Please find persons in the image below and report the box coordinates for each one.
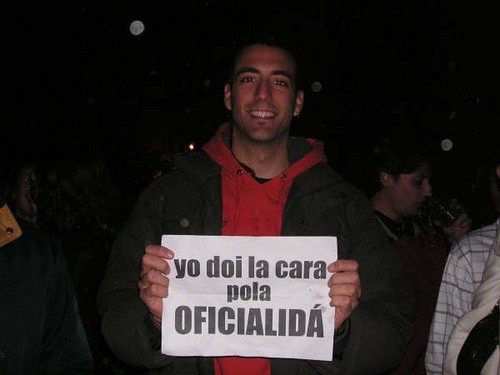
[96,28,416,375]
[363,135,472,375]
[0,201,95,375]
[424,218,500,375]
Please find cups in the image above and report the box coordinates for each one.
[424,196,458,228]
